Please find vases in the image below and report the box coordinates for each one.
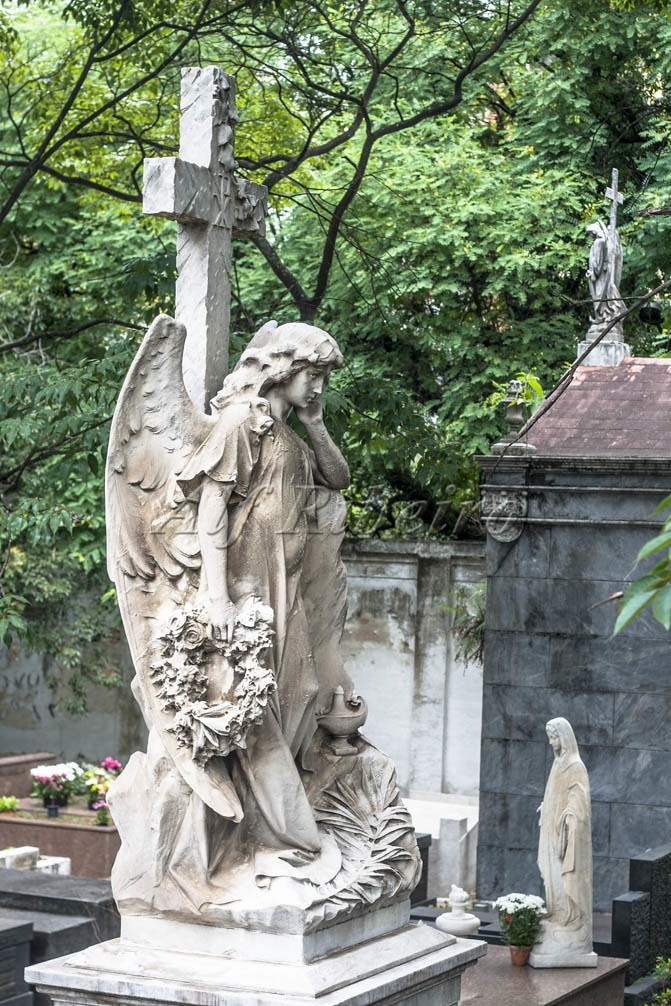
[44,793,68,809]
[510,945,532,968]
[88,800,96,809]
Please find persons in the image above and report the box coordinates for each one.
[587,221,622,321]
[175,321,353,876]
[536,716,593,954]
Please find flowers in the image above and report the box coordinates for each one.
[492,892,552,948]
[29,756,123,803]
